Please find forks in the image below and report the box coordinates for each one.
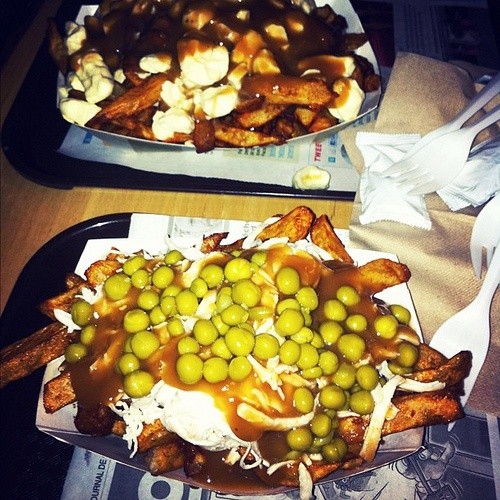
[469,192,500,279]
[428,249,500,432]
[380,79,500,195]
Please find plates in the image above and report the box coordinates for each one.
[56,0,381,149]
[34,238,423,493]
